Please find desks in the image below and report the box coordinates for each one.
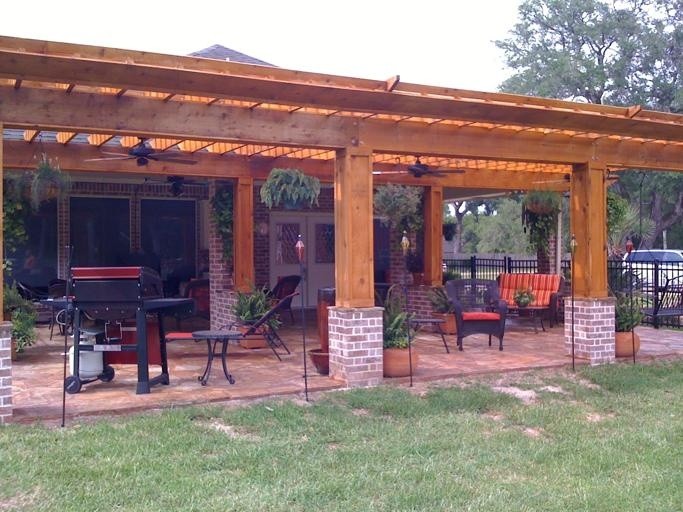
[39,294,72,340]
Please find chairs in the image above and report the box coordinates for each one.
[17,283,55,340]
[264,274,299,324]
[639,276,682,328]
[444,277,507,352]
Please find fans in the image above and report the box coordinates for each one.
[82,132,199,168]
[368,156,466,178]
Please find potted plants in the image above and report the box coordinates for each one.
[259,167,321,211]
[375,285,456,377]
[616,291,645,357]
[223,280,282,349]
[522,171,561,232]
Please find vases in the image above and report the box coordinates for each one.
[308,349,329,374]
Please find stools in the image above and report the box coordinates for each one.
[193,329,244,385]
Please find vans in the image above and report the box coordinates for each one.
[616,249,683,293]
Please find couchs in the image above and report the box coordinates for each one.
[493,273,562,329]
